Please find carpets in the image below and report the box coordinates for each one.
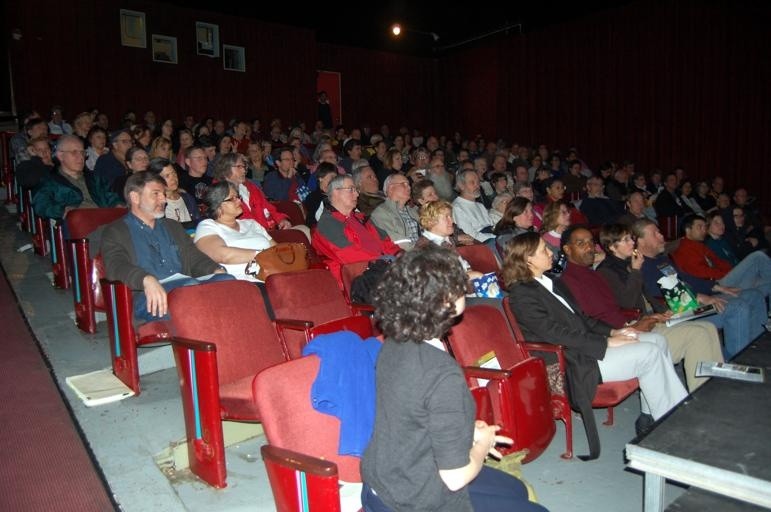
[1,262,124,512]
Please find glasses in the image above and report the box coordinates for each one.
[224,194,242,202]
[337,186,361,193]
[131,156,149,161]
[61,149,85,156]
[163,171,178,180]
[231,165,249,169]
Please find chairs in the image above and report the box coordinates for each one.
[48,217,71,290]
[253,343,376,512]
[30,202,49,257]
[67,207,129,335]
[340,261,375,316]
[167,280,313,489]
[457,244,504,288]
[92,226,173,394]
[0,136,30,229]
[502,295,639,459]
[447,305,573,465]
[265,268,376,360]
[270,200,305,226]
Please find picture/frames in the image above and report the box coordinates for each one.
[119,8,147,48]
[196,21,220,58]
[223,43,246,72]
[152,34,178,64]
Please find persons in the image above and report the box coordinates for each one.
[192,180,276,284]
[703,211,739,265]
[722,207,771,256]
[360,245,548,512]
[692,181,716,209]
[73,112,90,146]
[733,188,752,207]
[312,174,404,264]
[595,223,670,319]
[559,226,727,393]
[115,108,176,174]
[535,178,579,220]
[82,126,109,167]
[490,192,514,210]
[503,231,686,433]
[599,162,613,179]
[674,214,771,296]
[148,160,205,231]
[301,160,339,219]
[717,194,730,209]
[371,173,423,250]
[656,172,696,218]
[580,176,624,222]
[411,179,475,247]
[618,191,653,226]
[627,173,656,196]
[263,150,303,200]
[629,217,764,358]
[677,177,707,217]
[623,160,636,175]
[15,134,59,189]
[180,145,214,192]
[540,201,571,249]
[608,169,630,199]
[215,154,292,229]
[176,118,339,167]
[8,111,40,156]
[89,106,99,123]
[451,168,494,241]
[674,166,688,185]
[95,113,110,132]
[513,179,543,227]
[33,134,127,220]
[351,166,386,214]
[47,106,70,134]
[335,122,591,178]
[107,147,149,198]
[709,176,724,199]
[317,90,333,130]
[414,199,458,255]
[493,197,534,259]
[100,171,235,321]
[15,120,55,163]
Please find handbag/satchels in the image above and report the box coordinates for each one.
[483,448,538,503]
[350,257,392,316]
[245,241,311,281]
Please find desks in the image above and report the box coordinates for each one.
[626,329,770,512]
